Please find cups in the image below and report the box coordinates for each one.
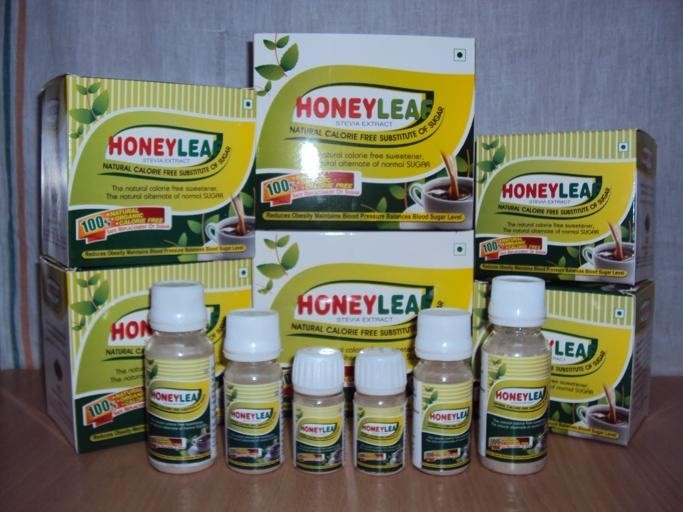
[205,215,256,260]
[575,404,629,445]
[582,241,635,284]
[408,176,474,230]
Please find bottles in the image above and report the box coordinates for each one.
[353,346,407,476]
[144,279,218,474]
[479,275,551,474]
[411,308,474,476]
[223,308,285,475]
[291,345,346,474]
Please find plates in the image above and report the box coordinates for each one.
[197,240,223,261]
[567,421,592,439]
[399,203,439,230]
[574,262,601,282]
